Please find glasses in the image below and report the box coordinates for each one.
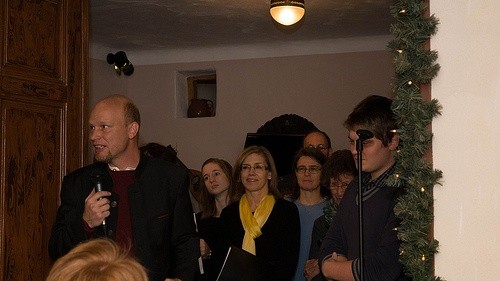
[296,166,320,174]
[329,182,349,190]
[241,163,270,172]
[306,144,329,152]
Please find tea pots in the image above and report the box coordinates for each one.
[187,99,214,118]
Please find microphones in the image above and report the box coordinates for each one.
[93,173,107,231]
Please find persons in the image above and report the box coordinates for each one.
[280,130,332,202]
[293,147,331,281]
[195,158,243,281]
[318,95,410,281]
[47,238,148,281]
[305,150,358,281]
[49,95,200,281]
[218,146,300,281]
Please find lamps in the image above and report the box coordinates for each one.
[269,0,306,27]
